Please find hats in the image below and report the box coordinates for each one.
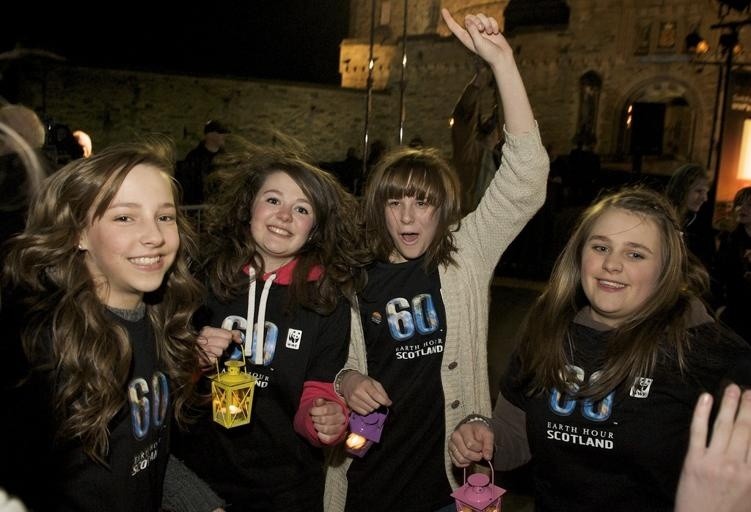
[203,118,232,134]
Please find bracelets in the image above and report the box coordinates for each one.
[335,369,351,392]
[463,419,486,425]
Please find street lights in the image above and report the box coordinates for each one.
[689,29,749,231]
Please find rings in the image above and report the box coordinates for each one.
[466,442,475,448]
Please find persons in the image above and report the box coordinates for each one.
[368,139,385,165]
[556,133,591,169]
[321,9,552,512]
[0,103,62,235]
[179,118,230,206]
[711,187,751,305]
[719,274,751,344]
[447,173,751,512]
[0,133,228,512]
[670,163,714,260]
[171,145,382,511]
